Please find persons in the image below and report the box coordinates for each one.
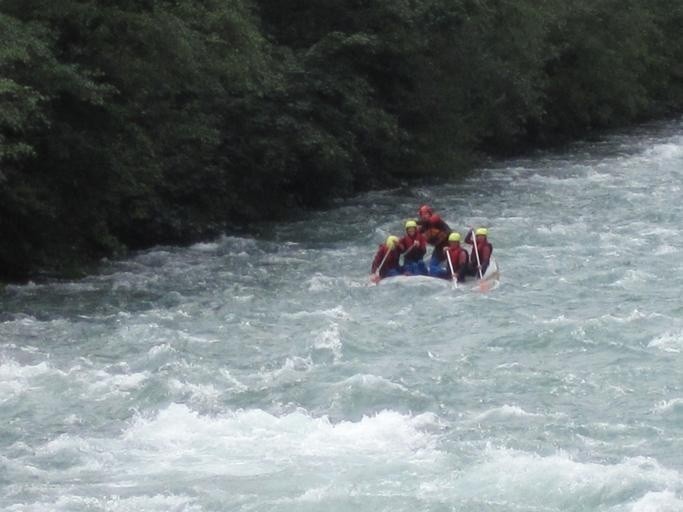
[372,205,493,282]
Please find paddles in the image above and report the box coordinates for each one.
[470,223,489,293]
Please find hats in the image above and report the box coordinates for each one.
[475,228,488,236]
[405,221,417,229]
[418,205,442,225]
[448,232,461,242]
[386,235,399,248]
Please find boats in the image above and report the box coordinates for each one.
[368,245,502,294]
